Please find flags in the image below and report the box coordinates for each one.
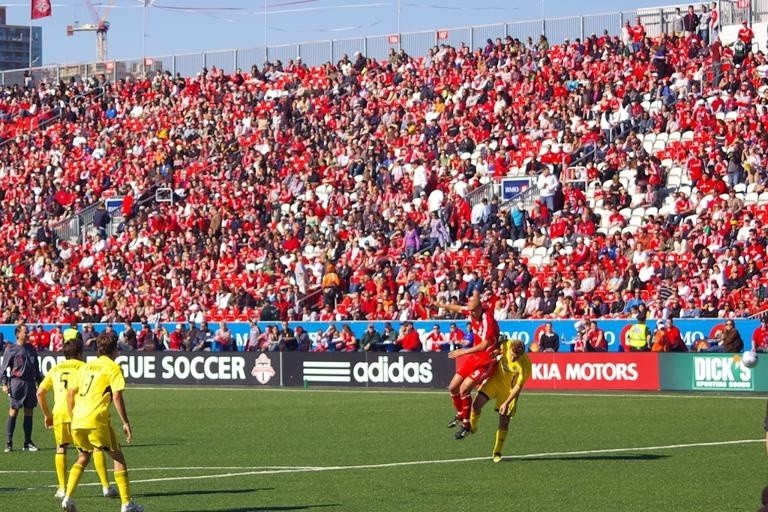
[32,0,51,20]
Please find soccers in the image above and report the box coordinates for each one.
[742,351,758,367]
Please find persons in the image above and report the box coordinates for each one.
[36,339,120,498]
[0,324,43,452]
[60,333,143,512]
[0,2,768,354]
[430,299,499,440]
[469,339,532,462]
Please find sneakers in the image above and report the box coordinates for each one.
[454,425,471,440]
[3,440,12,452]
[470,406,480,434]
[492,450,502,463]
[22,441,39,452]
[60,495,76,511]
[101,484,119,497]
[54,488,65,497]
[121,498,145,512]
[446,414,463,428]
